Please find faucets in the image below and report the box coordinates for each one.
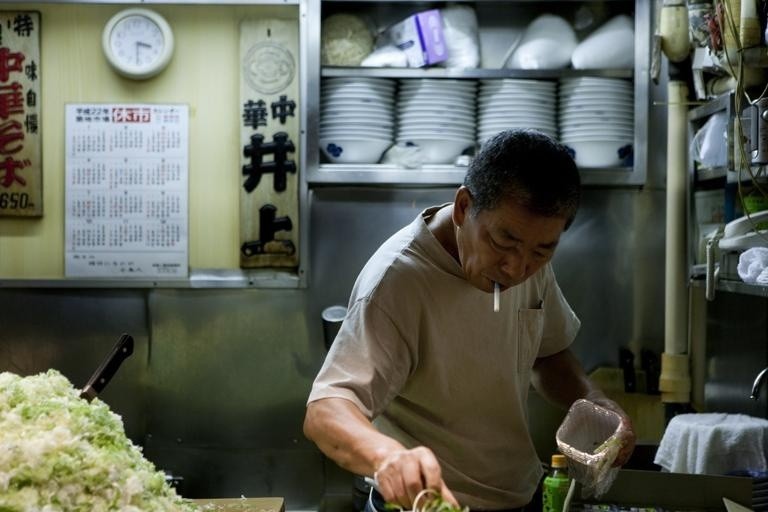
[748,368,767,394]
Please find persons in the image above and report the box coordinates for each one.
[303,126,638,510]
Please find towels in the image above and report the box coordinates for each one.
[653,412,768,475]
[738,248,768,286]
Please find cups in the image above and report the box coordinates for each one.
[554,396,624,497]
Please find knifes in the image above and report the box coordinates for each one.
[616,347,660,397]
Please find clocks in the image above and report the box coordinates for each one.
[103,8,175,81]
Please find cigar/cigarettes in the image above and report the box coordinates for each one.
[493,281,500,312]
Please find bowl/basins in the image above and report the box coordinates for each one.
[318,74,633,170]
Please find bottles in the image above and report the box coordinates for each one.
[543,455,577,512]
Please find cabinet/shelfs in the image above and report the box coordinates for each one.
[302,1,649,187]
[685,95,768,296]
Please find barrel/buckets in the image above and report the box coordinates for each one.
[390,10,450,67]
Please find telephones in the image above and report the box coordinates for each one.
[718,210,768,251]
